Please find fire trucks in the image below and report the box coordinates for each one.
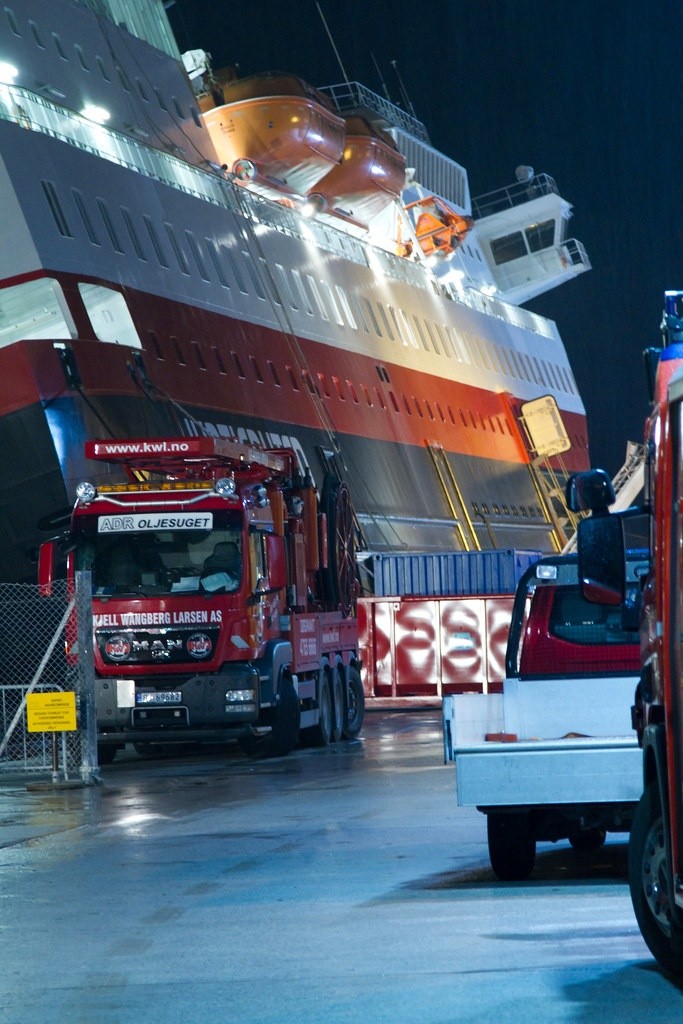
[38,434,370,776]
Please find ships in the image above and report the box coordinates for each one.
[0,0,587,713]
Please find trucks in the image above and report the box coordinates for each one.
[446,514,661,877]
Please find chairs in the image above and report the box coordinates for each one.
[203,541,241,578]
